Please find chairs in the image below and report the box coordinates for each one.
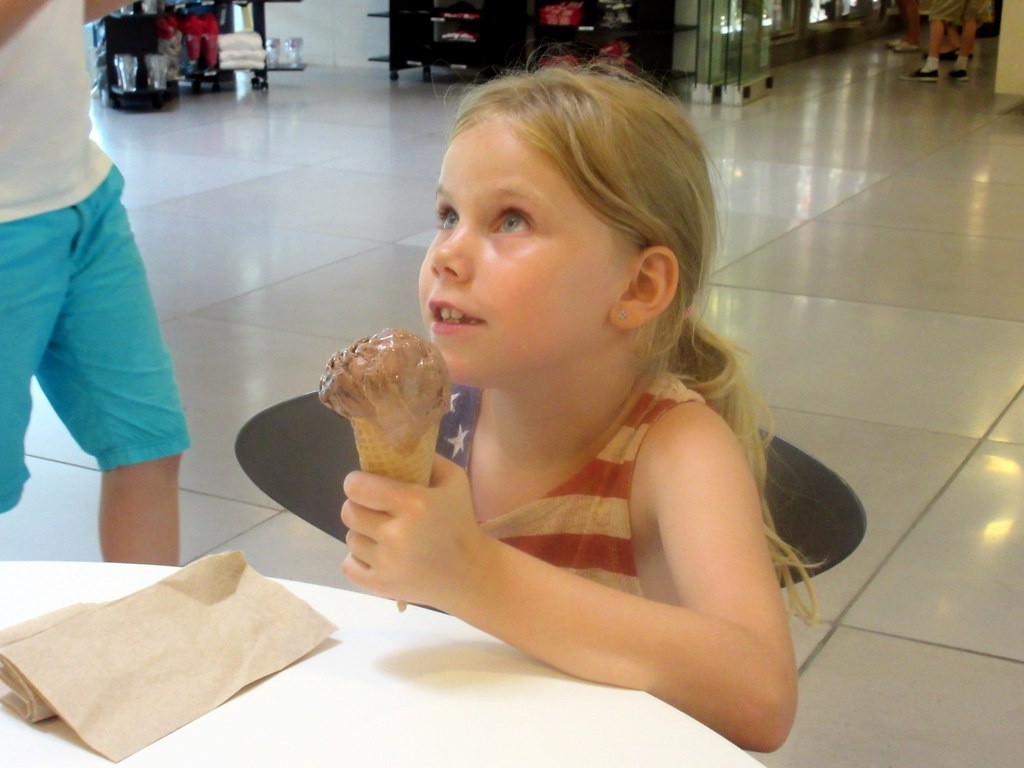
[232,388,866,594]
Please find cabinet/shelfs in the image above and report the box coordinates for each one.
[95,0,267,111]
[366,0,697,92]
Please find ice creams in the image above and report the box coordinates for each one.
[318,328,452,608]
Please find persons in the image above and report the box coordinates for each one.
[340,56,818,753]
[0,1,190,566]
[886,0,993,81]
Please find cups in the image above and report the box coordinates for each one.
[266,38,280,67]
[114,54,138,92]
[285,38,304,67]
[144,54,169,89]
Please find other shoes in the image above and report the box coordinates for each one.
[948,69,968,81]
[923,46,974,60]
[901,68,938,80]
[887,38,920,52]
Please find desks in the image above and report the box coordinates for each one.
[0,559,763,768]
